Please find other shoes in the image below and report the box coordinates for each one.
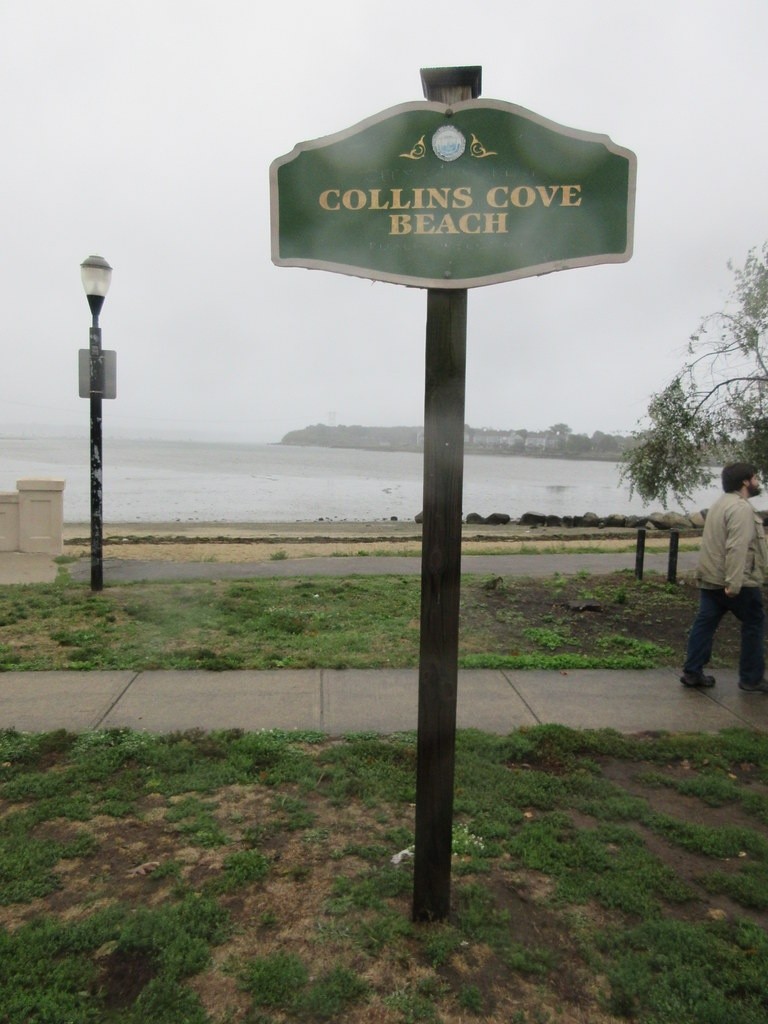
[680,670,716,687]
[738,679,768,693]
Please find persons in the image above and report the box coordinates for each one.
[680,463,768,692]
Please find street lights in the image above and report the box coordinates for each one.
[81,256,112,592]
